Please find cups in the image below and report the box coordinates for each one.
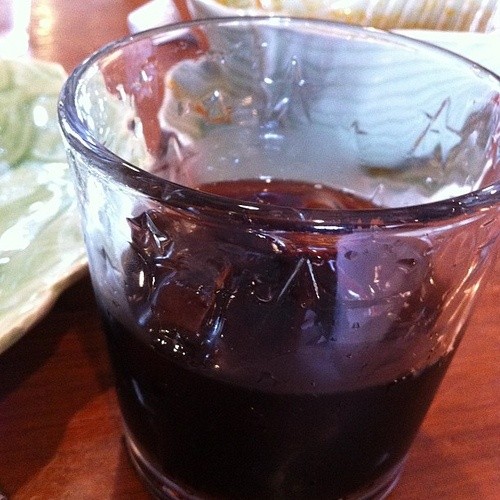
[59,16,500,500]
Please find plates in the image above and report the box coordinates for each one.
[0,55,91,355]
[127,0,194,35]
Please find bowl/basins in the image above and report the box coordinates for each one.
[192,1,500,62]
